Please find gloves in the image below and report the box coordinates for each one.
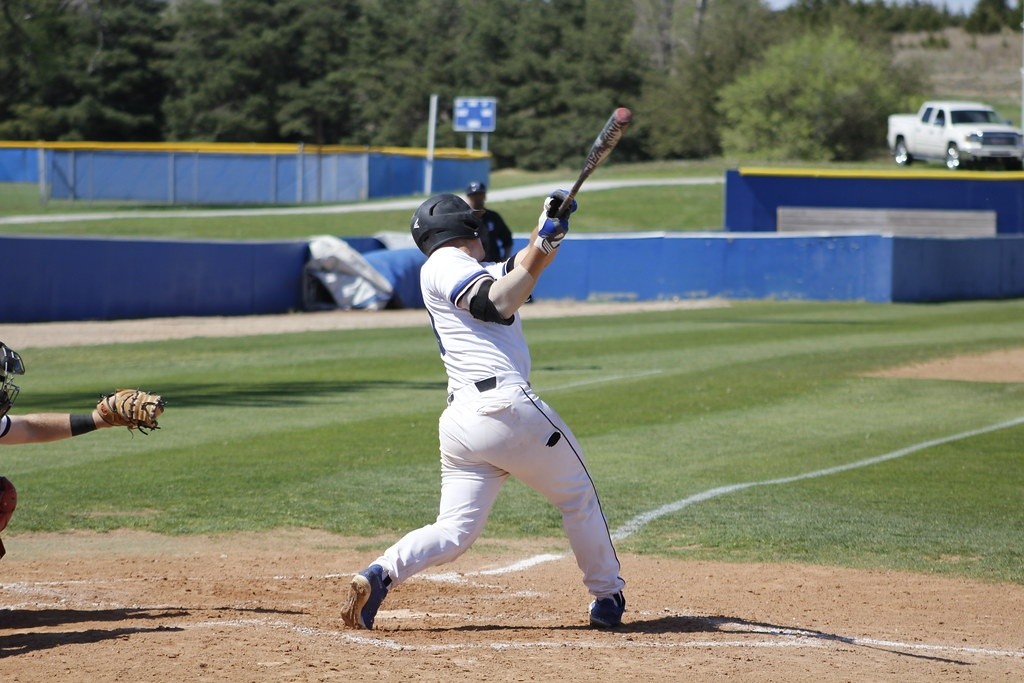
[538,208,569,256]
[542,188,578,217]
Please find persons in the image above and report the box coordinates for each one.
[0,337,166,560]
[339,193,626,631]
[465,181,514,261]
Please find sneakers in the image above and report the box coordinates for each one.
[341,564,388,633]
[585,597,623,627]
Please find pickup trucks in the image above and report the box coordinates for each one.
[887,99,1024,171]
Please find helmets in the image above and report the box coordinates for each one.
[412,192,485,257]
[0,343,28,417]
[467,183,489,195]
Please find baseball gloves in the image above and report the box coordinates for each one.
[96,389,165,431]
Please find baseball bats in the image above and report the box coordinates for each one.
[555,106,634,216]
